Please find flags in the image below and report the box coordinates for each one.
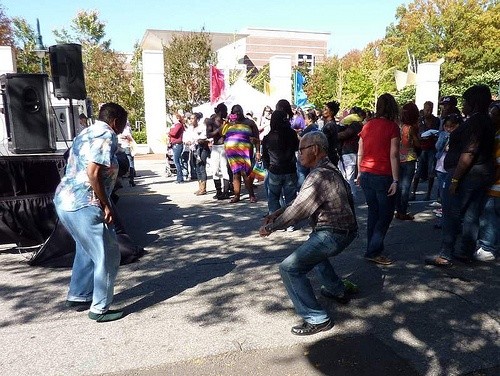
[211,66,225,102]
[296,71,307,105]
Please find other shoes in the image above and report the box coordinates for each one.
[194,190,206,195]
[87,310,125,322]
[230,197,239,203]
[395,213,399,219]
[429,201,444,228]
[250,195,257,203]
[65,299,93,309]
[399,215,415,220]
[213,191,230,200]
[174,180,184,184]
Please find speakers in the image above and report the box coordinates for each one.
[0,72,56,154]
[49,44,87,100]
[53,107,84,140]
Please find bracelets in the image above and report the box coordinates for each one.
[393,181,398,183]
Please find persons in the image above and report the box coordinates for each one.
[259,131,359,335]
[357,93,402,264]
[80,113,94,127]
[424,84,500,267]
[395,96,465,219]
[117,121,136,187]
[53,102,128,321]
[168,99,373,233]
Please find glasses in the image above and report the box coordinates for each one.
[297,144,315,155]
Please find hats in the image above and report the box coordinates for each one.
[439,97,457,106]
[327,100,340,116]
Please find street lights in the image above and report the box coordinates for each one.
[29,18,48,73]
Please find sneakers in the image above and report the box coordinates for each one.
[453,248,474,266]
[320,284,350,304]
[366,255,393,264]
[291,318,333,335]
[424,255,455,269]
[472,246,496,263]
